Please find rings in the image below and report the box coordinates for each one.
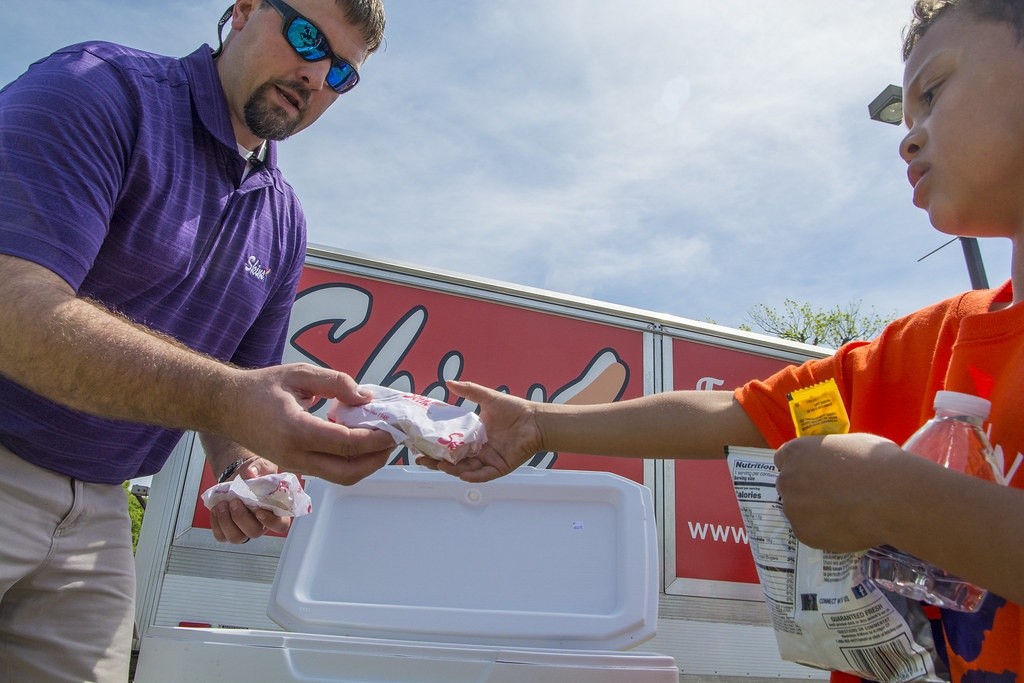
[241,537,250,544]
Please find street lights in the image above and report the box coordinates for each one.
[868,85,990,292]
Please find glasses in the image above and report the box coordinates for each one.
[266,0,360,94]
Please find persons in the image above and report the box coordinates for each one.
[415,0,1023,683]
[0,0,398,683]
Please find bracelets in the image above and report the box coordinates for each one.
[217,455,257,484]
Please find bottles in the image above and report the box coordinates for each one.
[858,388,1002,614]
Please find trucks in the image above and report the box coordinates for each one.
[132,242,840,683]
